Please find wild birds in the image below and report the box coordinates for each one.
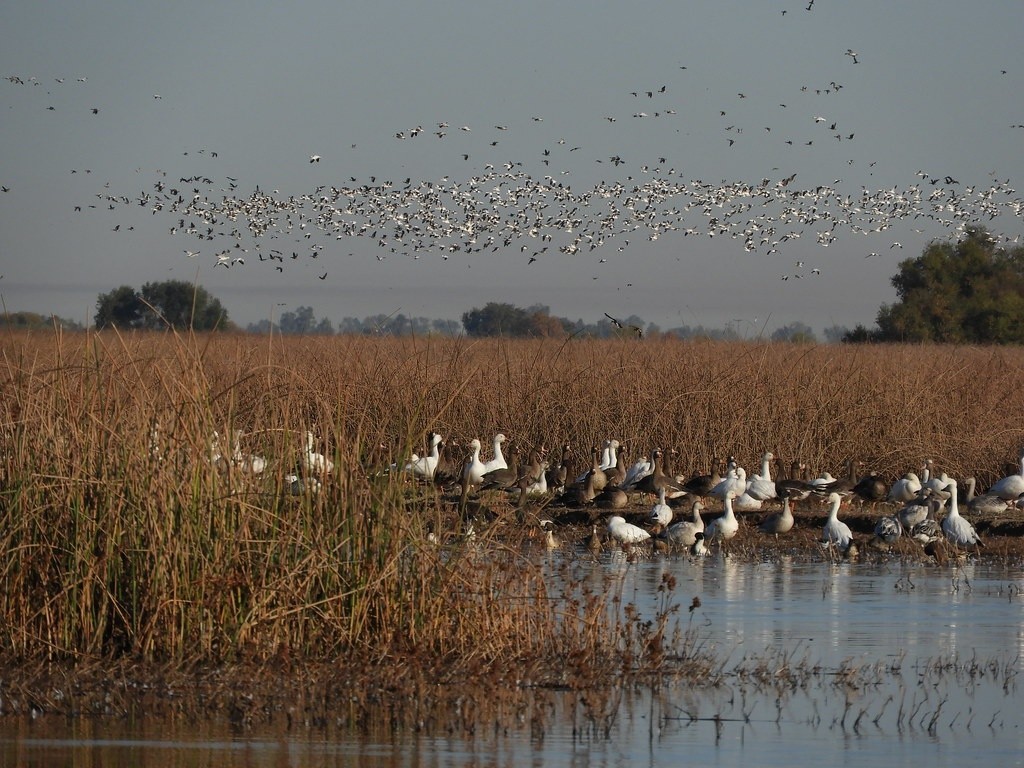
[133,411,1023,566]
[0,2,1023,340]
